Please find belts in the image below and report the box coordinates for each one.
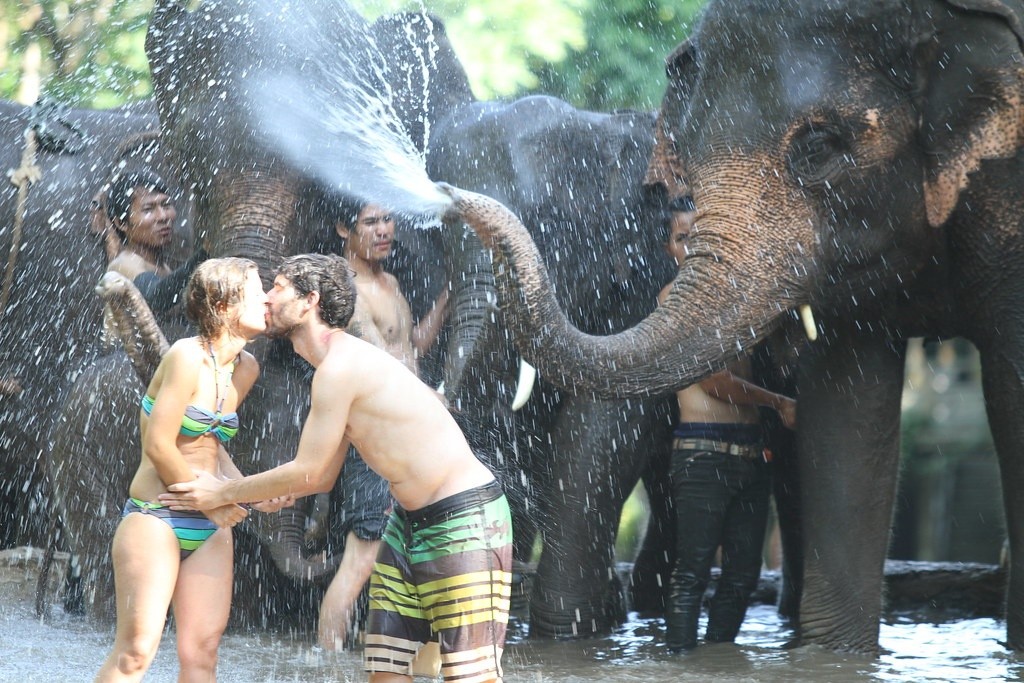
[673,438,760,458]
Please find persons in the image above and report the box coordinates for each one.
[655,193,797,655]
[316,197,451,650]
[97,254,296,683]
[101,166,178,352]
[157,252,514,683]
